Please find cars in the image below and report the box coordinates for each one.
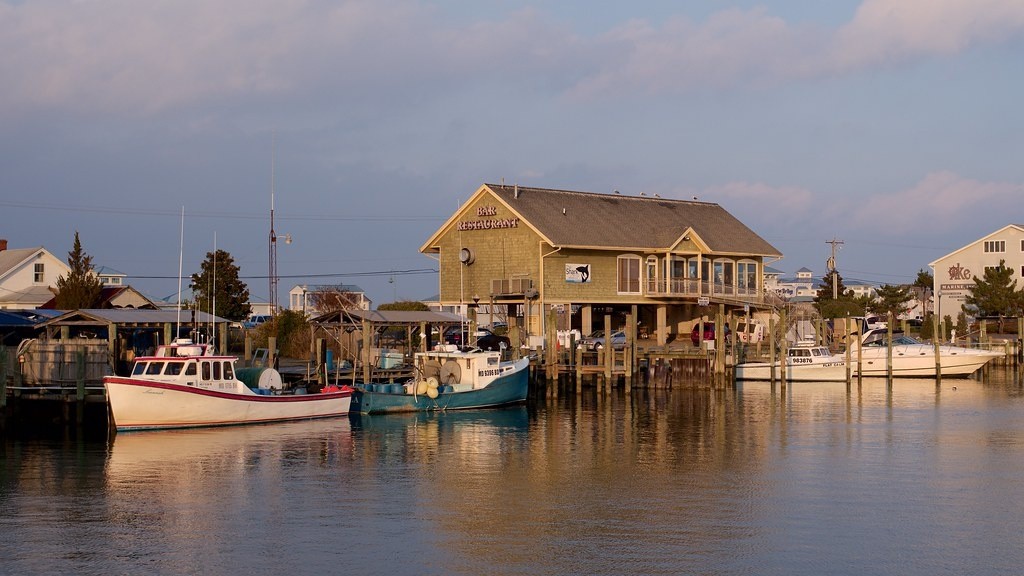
[691,321,739,345]
[574,328,629,353]
[915,314,924,324]
[444,327,511,353]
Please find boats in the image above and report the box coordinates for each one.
[842,328,1008,379]
[330,342,531,415]
[101,350,355,433]
[726,344,859,384]
